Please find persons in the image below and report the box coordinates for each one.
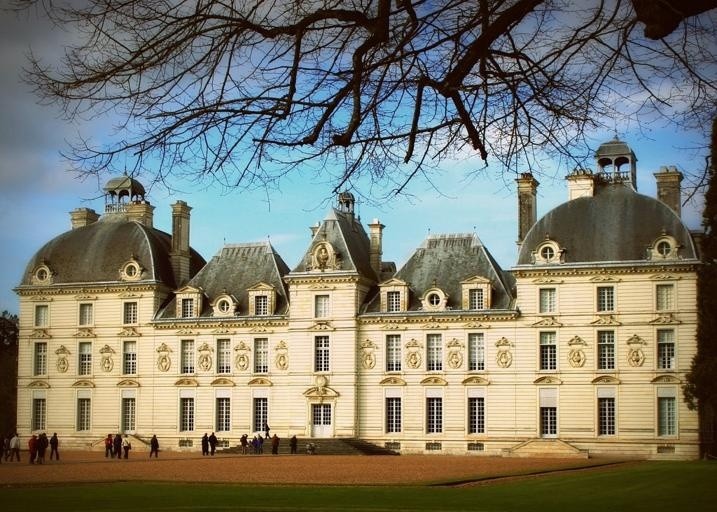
[28,432,59,464]
[201,433,218,456]
[149,435,159,458]
[3,433,20,462]
[239,433,297,455]
[264,423,271,439]
[105,433,131,459]
